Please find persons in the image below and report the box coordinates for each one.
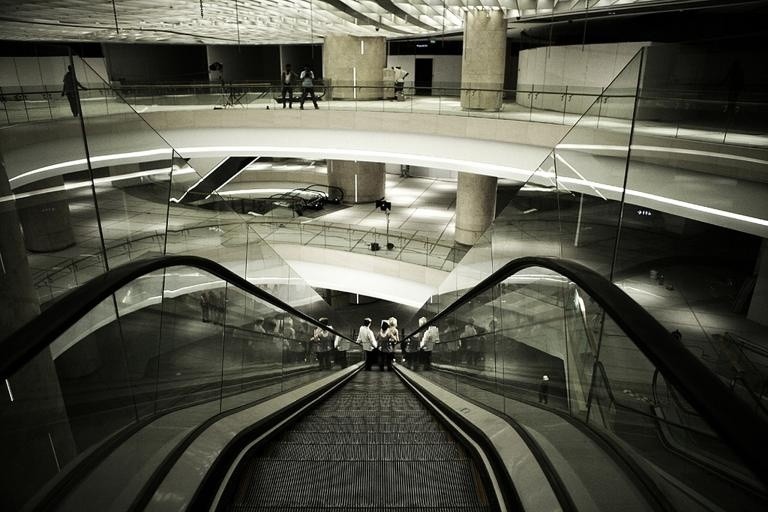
[314,318,333,371]
[62,65,88,117]
[356,316,399,371]
[281,63,294,108]
[209,60,224,93]
[300,67,319,110]
[538,375,549,404]
[254,317,309,370]
[200,291,230,323]
[391,65,409,100]
[334,335,350,369]
[405,317,507,371]
